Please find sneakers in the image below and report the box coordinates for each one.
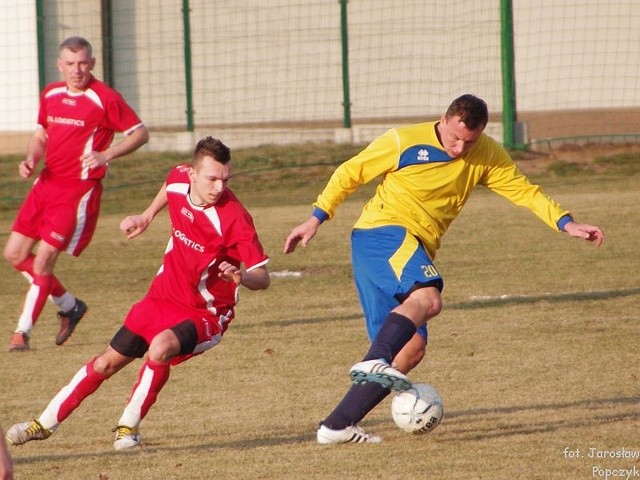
[56,297,87,346]
[350,358,413,392]
[8,330,29,353]
[111,426,142,451]
[316,421,384,445]
[6,419,53,446]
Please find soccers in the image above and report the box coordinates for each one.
[392,384,444,434]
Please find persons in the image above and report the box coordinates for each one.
[0,423,15,479]
[284,95,604,446]
[4,36,150,349]
[5,135,270,452]
